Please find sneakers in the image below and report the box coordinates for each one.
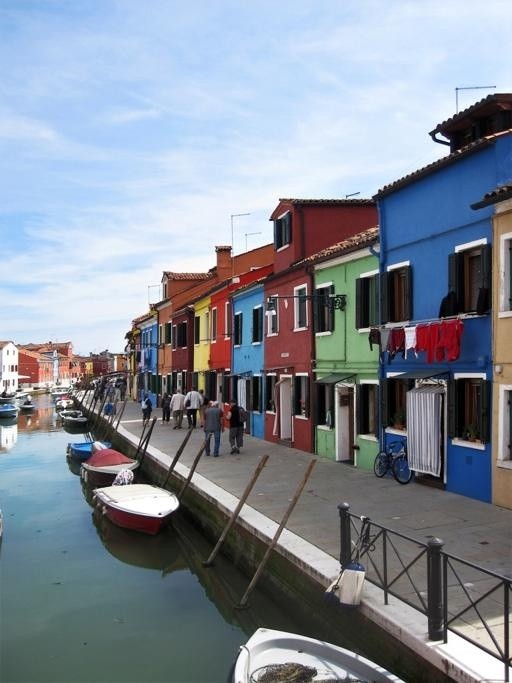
[230,447,240,454]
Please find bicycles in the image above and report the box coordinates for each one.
[373,438,413,485]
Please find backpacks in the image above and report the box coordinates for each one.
[141,398,149,410]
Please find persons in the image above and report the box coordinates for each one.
[136,384,213,430]
[223,398,246,454]
[92,375,127,403]
[202,400,225,457]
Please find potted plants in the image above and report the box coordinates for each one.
[466,424,478,442]
[393,409,405,429]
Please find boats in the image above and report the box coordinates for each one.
[0,392,35,419]
[50,386,89,429]
[66,441,181,537]
[232,626,407,683]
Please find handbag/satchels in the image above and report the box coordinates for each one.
[239,408,249,423]
[185,399,192,407]
